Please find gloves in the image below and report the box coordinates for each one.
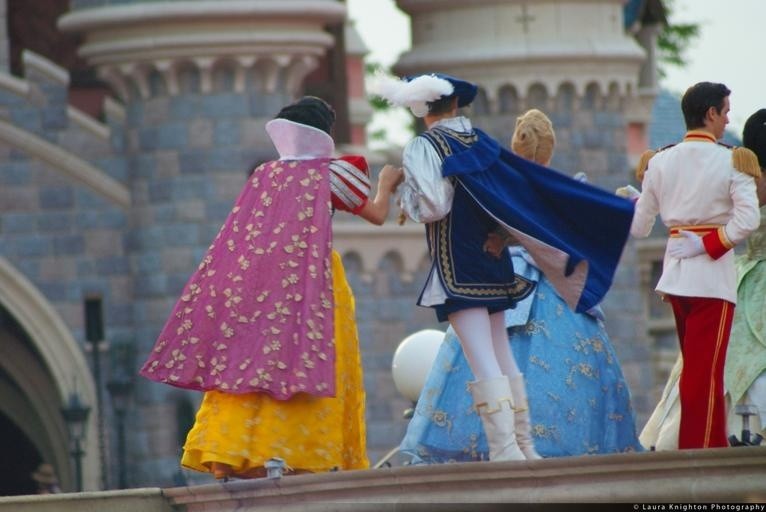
[667,229,707,260]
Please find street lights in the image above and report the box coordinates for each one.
[104,368,132,489]
[62,368,91,489]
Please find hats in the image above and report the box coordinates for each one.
[405,74,477,107]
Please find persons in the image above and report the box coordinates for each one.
[131,67,766,483]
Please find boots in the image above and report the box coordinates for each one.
[469,374,526,463]
[509,373,544,460]
